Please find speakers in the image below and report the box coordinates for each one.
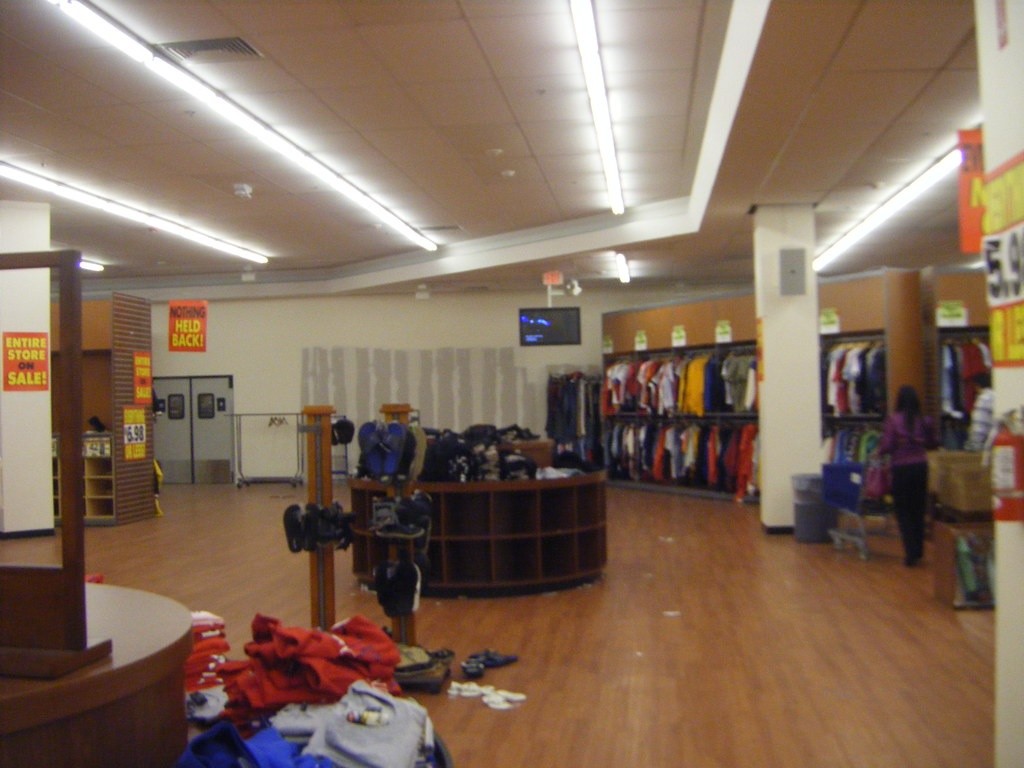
[779,248,807,296]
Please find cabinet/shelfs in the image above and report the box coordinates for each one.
[350,438,608,598]
[53,433,116,527]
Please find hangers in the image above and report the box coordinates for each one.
[607,411,884,434]
[604,332,989,365]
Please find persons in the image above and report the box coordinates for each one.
[873,385,938,567]
[963,373,993,451]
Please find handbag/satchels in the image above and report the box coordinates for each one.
[866,455,893,499]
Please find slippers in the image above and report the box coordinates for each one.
[382,421,405,474]
[301,504,320,551]
[284,505,302,551]
[358,422,383,475]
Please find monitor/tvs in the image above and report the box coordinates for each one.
[518,307,582,346]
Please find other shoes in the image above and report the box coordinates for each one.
[376,521,424,538]
[375,560,421,617]
[906,551,921,566]
